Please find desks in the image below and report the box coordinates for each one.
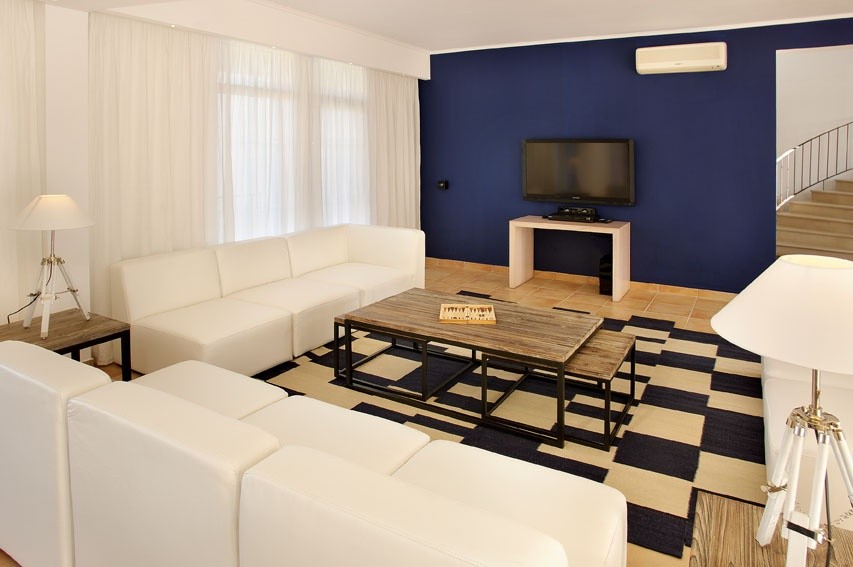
[0,310,132,380]
[689,491,852,567]
[332,285,641,449]
[509,215,636,301]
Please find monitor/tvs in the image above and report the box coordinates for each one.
[522,138,635,223]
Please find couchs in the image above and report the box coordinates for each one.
[113,220,429,374]
[0,340,630,567]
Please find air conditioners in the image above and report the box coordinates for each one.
[635,42,728,74]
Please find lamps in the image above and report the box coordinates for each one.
[709,254,853,566]
[11,194,105,338]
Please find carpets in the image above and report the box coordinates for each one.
[248,291,771,559]
